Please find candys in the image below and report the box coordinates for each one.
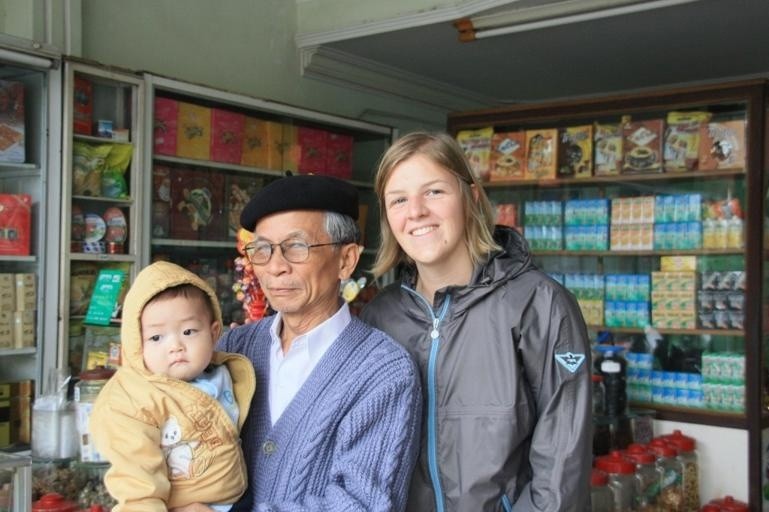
[230,227,271,329]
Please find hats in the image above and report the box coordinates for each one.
[238,169,361,238]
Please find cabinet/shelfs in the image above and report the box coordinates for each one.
[445,76,769,512]
[0,43,61,511]
[58,54,143,406]
[137,68,396,340]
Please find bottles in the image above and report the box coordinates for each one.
[30,364,117,464]
[590,344,701,512]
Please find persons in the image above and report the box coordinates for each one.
[93,260,258,511]
[216,166,425,512]
[357,131,593,512]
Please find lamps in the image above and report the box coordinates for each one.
[450,1,684,43]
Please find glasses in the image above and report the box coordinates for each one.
[240,238,354,266]
